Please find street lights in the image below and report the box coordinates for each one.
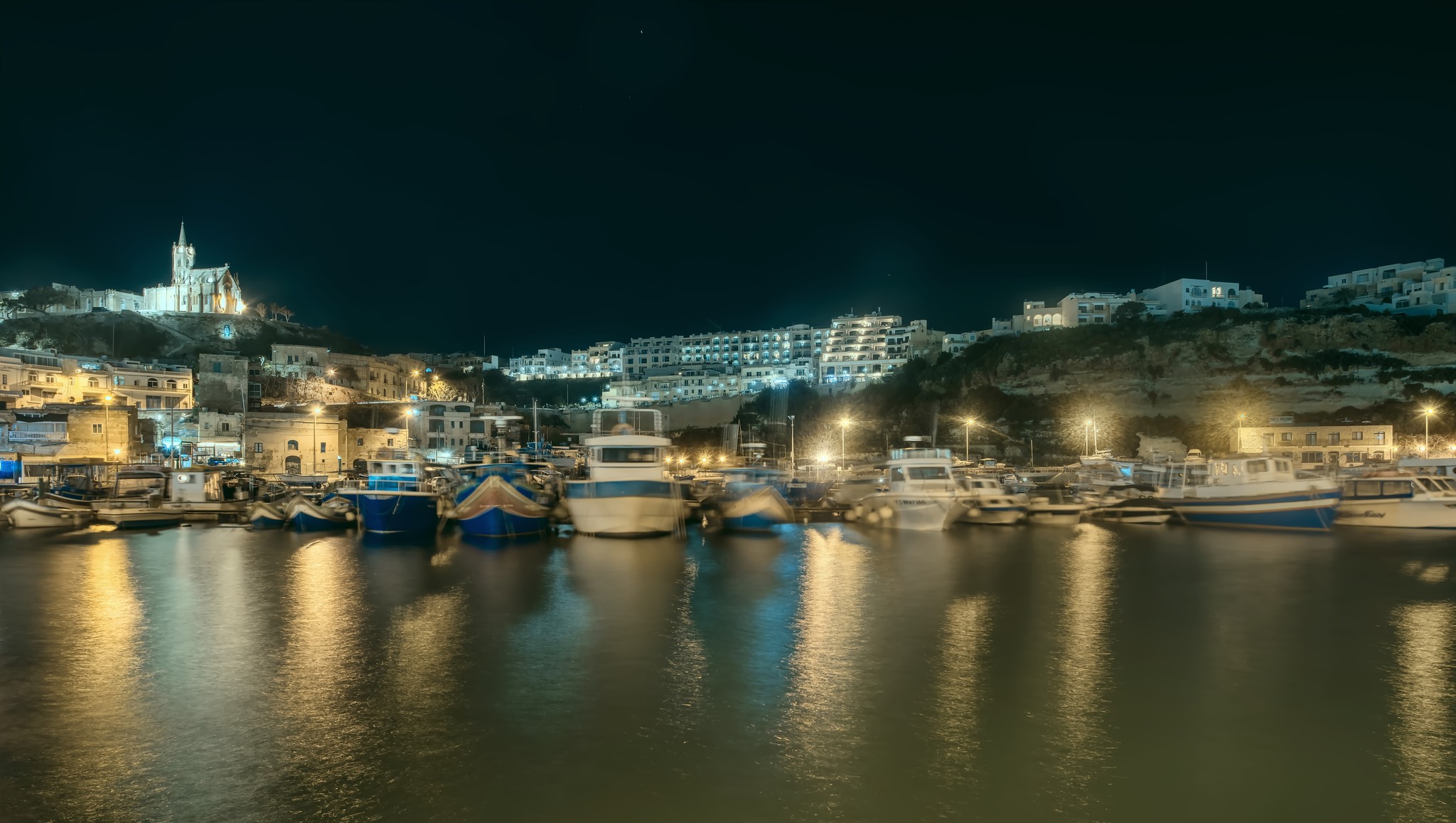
[966,419,974,462]
[105,396,111,461]
[1086,420,1091,455]
[1426,408,1433,459]
[406,410,411,450]
[1239,413,1244,428]
[314,408,320,471]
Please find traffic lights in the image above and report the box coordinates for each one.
[690,460,796,537]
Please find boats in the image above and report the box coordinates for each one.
[558,406,707,545]
[0,451,885,544]
[823,421,1456,536]
